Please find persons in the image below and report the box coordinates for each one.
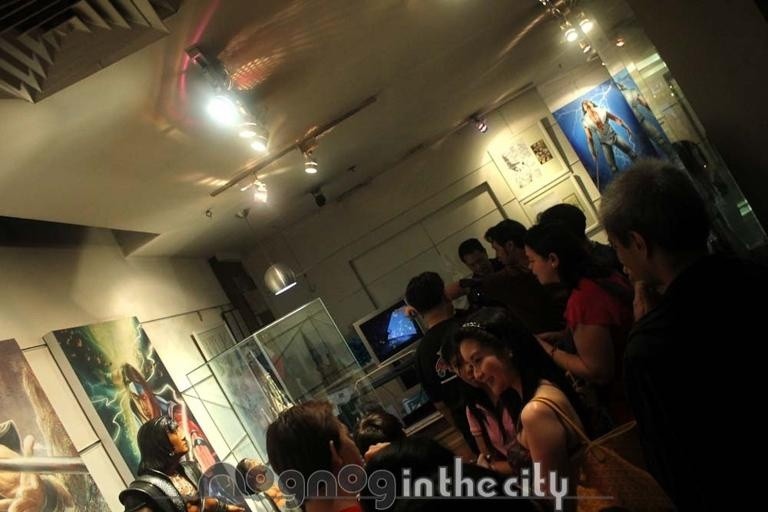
[351,407,403,455]
[119,412,247,511]
[360,435,456,512]
[265,398,392,511]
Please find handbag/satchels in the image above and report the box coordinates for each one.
[527,396,678,512]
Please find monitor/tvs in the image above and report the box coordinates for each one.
[352,295,426,368]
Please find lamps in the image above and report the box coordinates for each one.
[299,137,320,175]
[547,1,593,42]
[235,207,298,296]
